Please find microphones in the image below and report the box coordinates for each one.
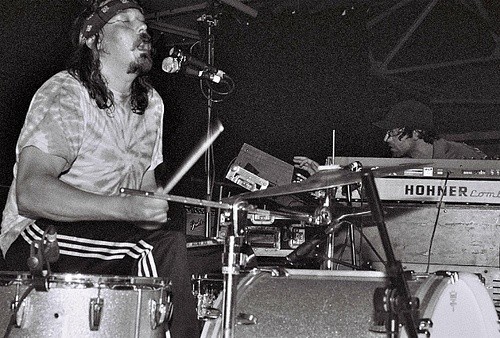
[162,57,221,83]
[169,46,226,80]
[44,226,60,263]
[281,223,340,268]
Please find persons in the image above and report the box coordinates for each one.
[294,101,487,175]
[0,0,260,338]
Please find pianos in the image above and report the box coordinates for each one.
[325,156,500,205]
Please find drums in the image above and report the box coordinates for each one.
[0,270,173,338]
[200,266,500,338]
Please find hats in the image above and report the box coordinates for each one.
[371,100,434,129]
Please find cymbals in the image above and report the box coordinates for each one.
[234,162,433,199]
